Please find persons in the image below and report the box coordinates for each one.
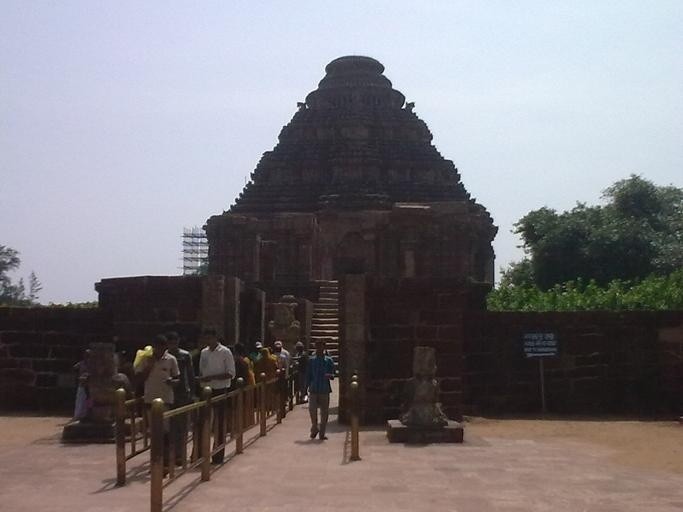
[304,339,335,441]
[69,335,310,469]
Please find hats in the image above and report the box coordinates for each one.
[295,340,304,347]
[273,340,282,348]
[255,341,262,349]
